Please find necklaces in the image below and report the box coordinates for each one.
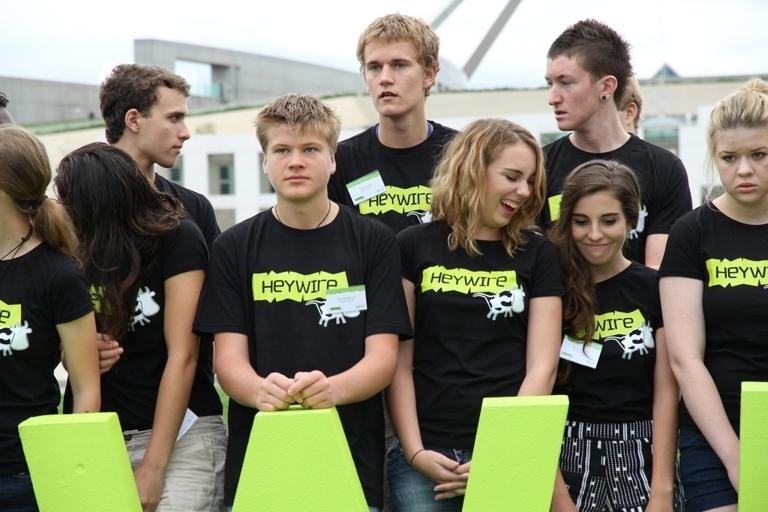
[275,199,331,230]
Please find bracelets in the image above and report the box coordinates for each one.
[410,449,425,467]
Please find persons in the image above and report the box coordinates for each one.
[527,18,693,271]
[55,143,227,512]
[0,126,102,512]
[616,74,643,137]
[192,93,414,509]
[328,13,462,235]
[548,160,687,512]
[387,118,564,512]
[0,91,16,125]
[62,64,222,375]
[658,76,768,512]
